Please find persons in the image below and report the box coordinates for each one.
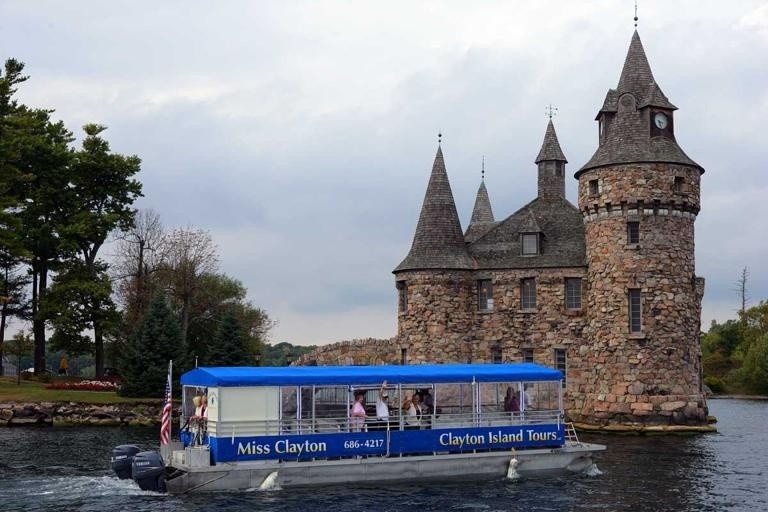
[519,384,536,410]
[61,355,69,376]
[421,394,434,430]
[193,395,210,421]
[503,386,520,419]
[376,380,388,431]
[350,393,366,433]
[190,396,203,416]
[403,393,424,431]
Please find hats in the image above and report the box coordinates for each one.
[382,392,389,398]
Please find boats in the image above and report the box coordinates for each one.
[111,363,606,497]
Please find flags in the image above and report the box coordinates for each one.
[159,363,173,446]
